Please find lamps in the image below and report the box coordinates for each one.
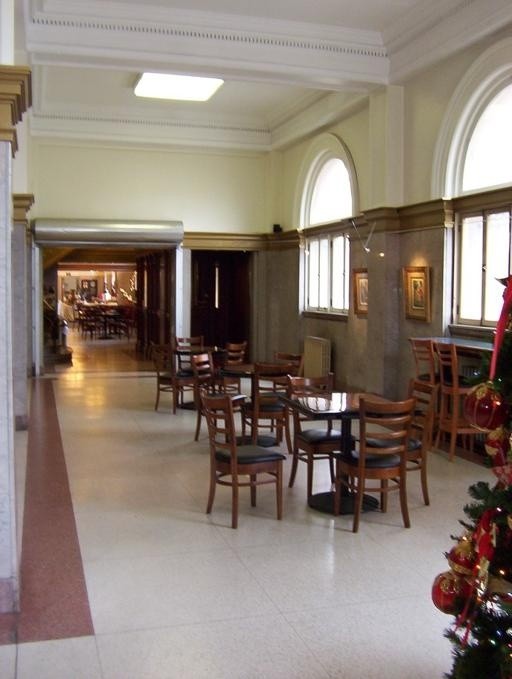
[132,73,224,101]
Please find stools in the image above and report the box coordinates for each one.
[406,337,491,463]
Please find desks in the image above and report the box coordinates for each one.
[408,336,494,373]
[278,389,394,515]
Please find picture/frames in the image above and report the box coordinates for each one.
[352,268,368,314]
[400,266,432,324]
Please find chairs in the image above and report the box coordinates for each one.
[286,371,356,499]
[149,336,305,447]
[334,396,416,532]
[198,387,288,530]
[72,303,133,341]
[360,374,441,514]
